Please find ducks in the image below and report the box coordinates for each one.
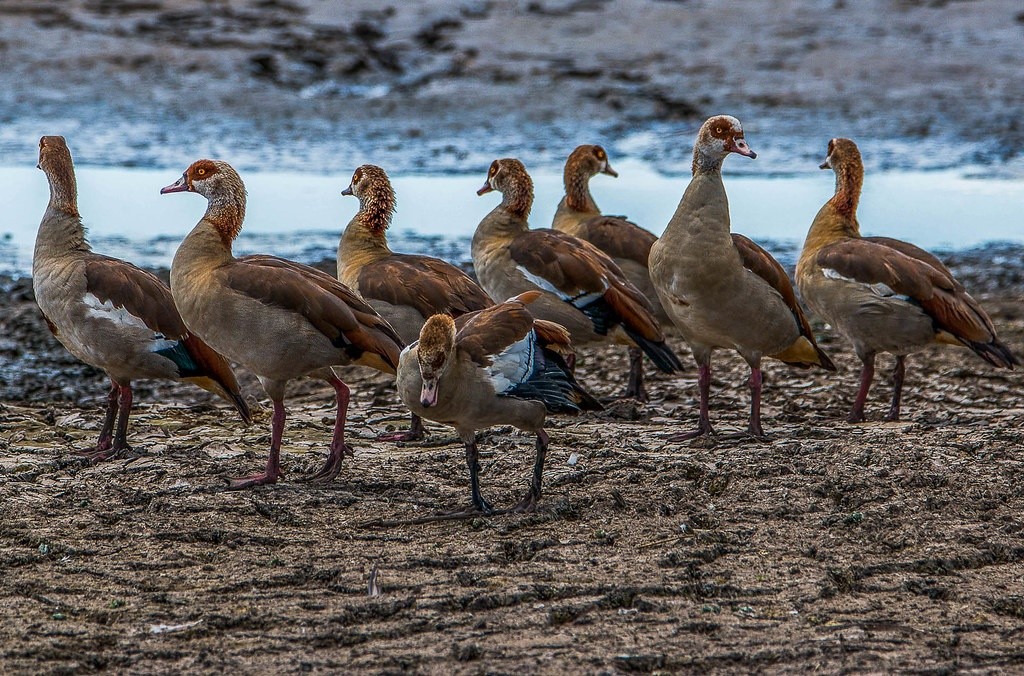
[397,290,607,515]
[793,138,1023,427]
[647,116,837,448]
[471,158,688,376]
[31,136,251,465]
[159,159,407,491]
[550,144,660,404]
[336,164,575,440]
